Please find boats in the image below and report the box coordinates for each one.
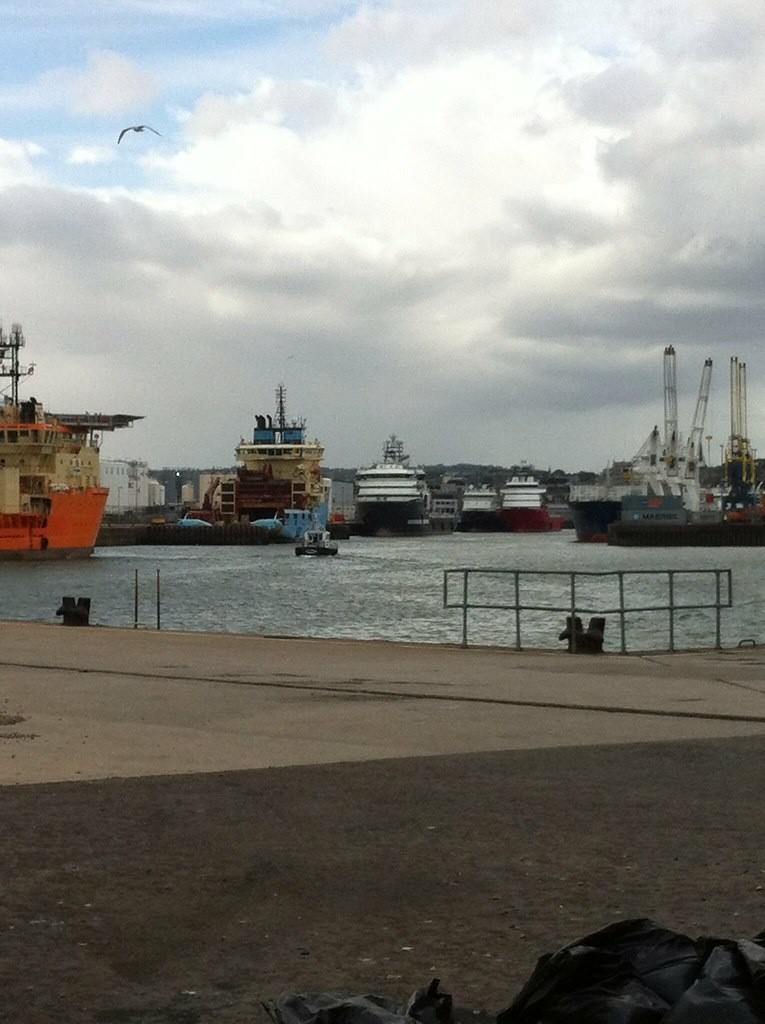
[494,476,563,532]
[0,324,109,562]
[178,381,333,543]
[352,434,464,538]
[295,526,339,557]
[566,424,725,543]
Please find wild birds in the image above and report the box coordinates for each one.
[116,124,163,145]
